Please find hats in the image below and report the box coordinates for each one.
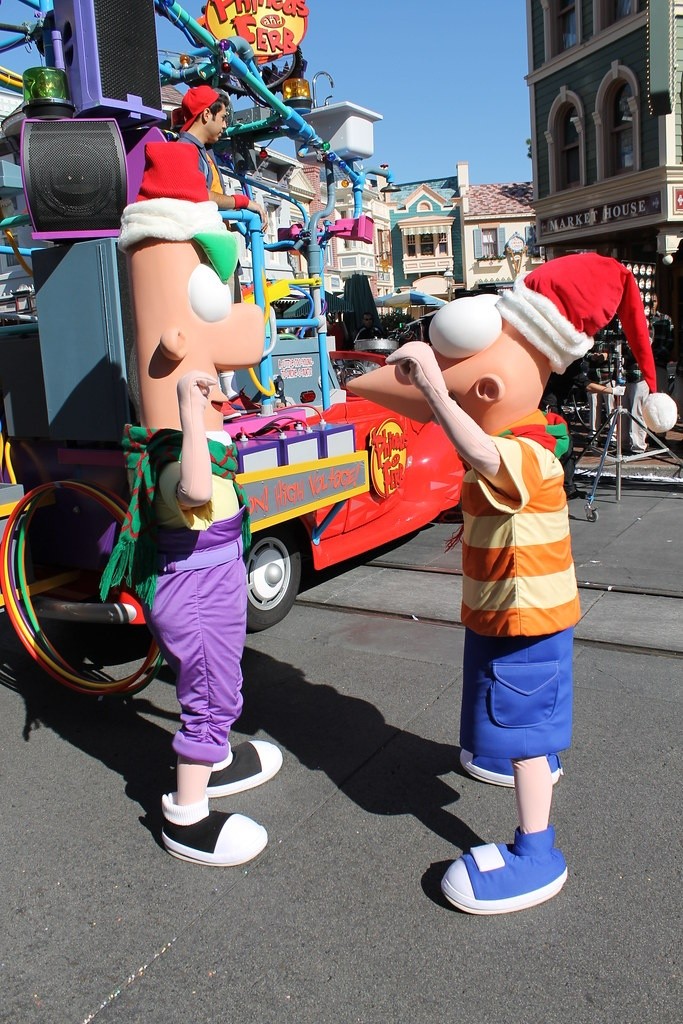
[495,253,677,433]
[180,85,220,131]
[118,142,227,254]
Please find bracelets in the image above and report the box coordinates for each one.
[232,194,249,209]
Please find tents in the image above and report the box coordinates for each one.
[282,291,353,322]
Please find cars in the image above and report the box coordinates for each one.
[19,350,467,635]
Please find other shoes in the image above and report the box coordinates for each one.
[206,741,282,798]
[460,747,564,788]
[441,826,568,915]
[162,791,268,866]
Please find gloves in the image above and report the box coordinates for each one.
[613,386,626,396]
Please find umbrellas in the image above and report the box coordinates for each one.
[342,273,381,351]
[374,287,450,316]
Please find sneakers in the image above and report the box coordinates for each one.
[227,387,262,414]
[622,451,641,458]
[211,401,241,420]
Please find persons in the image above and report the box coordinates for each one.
[344,252,678,914]
[539,295,675,500]
[352,313,385,342]
[98,143,284,869]
[177,86,268,422]
